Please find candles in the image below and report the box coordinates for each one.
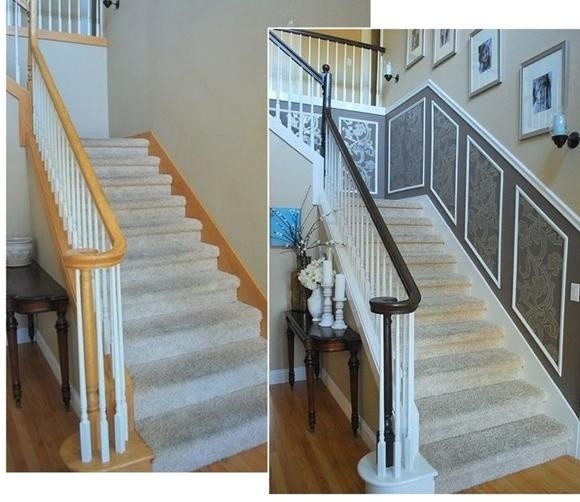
[335,274,345,299]
[322,260,332,285]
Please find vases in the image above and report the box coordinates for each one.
[6,236,33,268]
[307,289,324,322]
[291,250,312,312]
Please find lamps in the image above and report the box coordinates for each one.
[103,0,120,9]
[384,63,399,82]
[552,114,580,149]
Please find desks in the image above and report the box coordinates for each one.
[6,259,70,412]
[284,310,362,438]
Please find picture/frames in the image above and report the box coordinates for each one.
[519,40,569,141]
[404,29,425,70]
[431,29,456,68]
[467,29,502,98]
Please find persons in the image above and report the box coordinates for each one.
[478,44,490,71]
[533,75,550,114]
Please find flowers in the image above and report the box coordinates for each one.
[270,184,348,257]
[298,257,337,291]
